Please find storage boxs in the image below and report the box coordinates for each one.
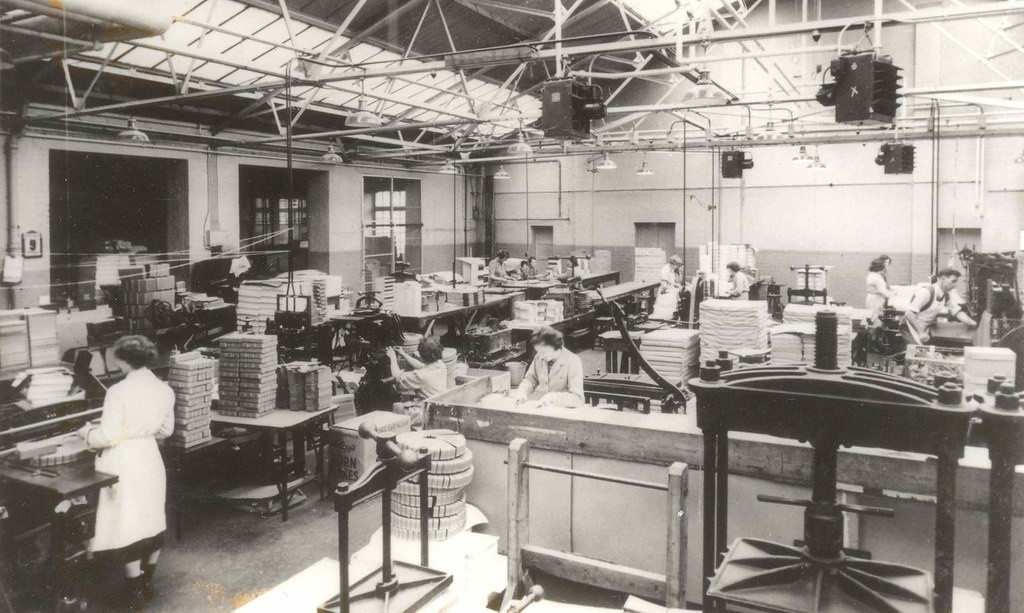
[964,345,1016,394]
[327,410,412,497]
[456,368,510,396]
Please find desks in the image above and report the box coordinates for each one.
[588,281,661,316]
[211,404,340,522]
[378,294,510,364]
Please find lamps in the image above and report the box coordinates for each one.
[793,133,814,161]
[115,110,150,142]
[596,152,617,169]
[805,143,826,169]
[679,40,731,105]
[345,77,382,127]
[507,119,533,153]
[438,158,457,173]
[636,152,653,175]
[493,164,510,179]
[320,136,343,163]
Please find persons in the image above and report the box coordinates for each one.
[865,254,977,365]
[487,249,517,288]
[511,325,586,409]
[73,335,176,608]
[657,254,690,294]
[386,336,447,423]
[724,261,750,301]
[518,256,550,281]
[554,256,586,290]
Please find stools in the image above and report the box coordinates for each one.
[592,317,615,351]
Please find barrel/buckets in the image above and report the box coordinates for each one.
[502,361,528,386]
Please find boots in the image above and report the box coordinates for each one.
[123,563,155,611]
